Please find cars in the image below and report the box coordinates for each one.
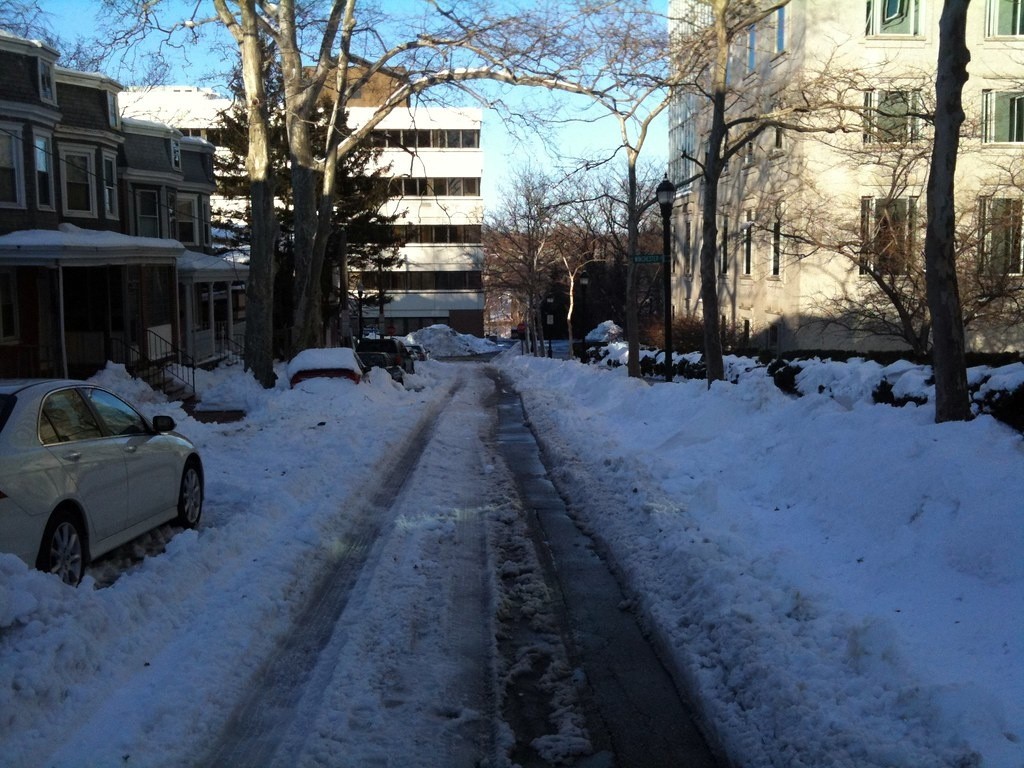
[290,338,431,393]
[0,380,204,589]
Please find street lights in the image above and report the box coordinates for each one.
[655,172,676,383]
